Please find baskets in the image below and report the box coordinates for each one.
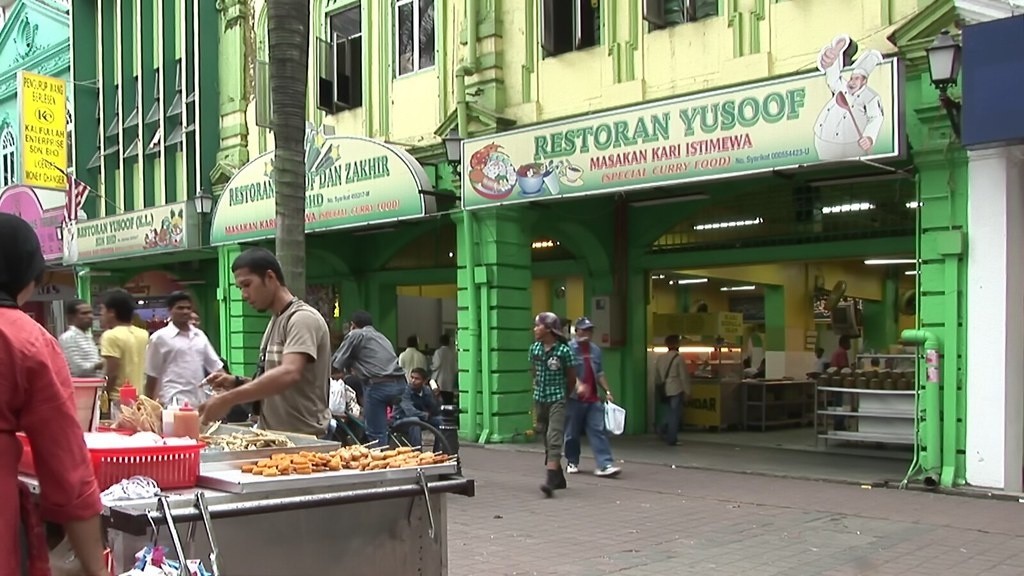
[14,427,134,476]
[82,429,207,491]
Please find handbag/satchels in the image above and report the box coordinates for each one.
[252,369,265,416]
[655,382,672,405]
[603,400,626,435]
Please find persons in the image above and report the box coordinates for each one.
[0,211,110,576]
[429,335,457,405]
[827,336,852,431]
[390,366,445,453]
[563,317,621,478]
[398,336,428,385]
[196,246,331,443]
[528,312,577,497]
[331,310,406,449]
[98,287,150,424]
[142,290,226,412]
[657,334,690,444]
[60,299,104,380]
[742,359,758,379]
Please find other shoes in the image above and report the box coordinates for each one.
[671,441,683,447]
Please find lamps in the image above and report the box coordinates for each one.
[193,186,215,226]
[55,224,62,240]
[925,29,972,136]
[443,130,465,182]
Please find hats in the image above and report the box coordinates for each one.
[575,317,596,330]
[539,312,564,336]
[0,212,45,296]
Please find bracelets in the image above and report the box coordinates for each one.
[235,375,245,387]
[605,390,612,395]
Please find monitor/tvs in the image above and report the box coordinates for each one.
[832,306,852,329]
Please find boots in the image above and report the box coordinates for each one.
[540,469,558,497]
[550,470,567,489]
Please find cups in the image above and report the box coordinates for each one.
[429,379,438,391]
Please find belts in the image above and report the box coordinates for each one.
[368,375,404,385]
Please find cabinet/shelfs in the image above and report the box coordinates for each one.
[742,380,829,434]
[655,340,747,434]
[815,386,916,447]
[855,355,917,374]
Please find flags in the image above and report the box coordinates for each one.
[61,176,91,238]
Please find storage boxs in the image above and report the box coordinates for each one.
[73,376,109,432]
[14,433,207,492]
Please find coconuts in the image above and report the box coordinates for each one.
[817,367,916,390]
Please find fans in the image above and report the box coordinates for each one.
[815,277,847,309]
[694,302,708,312]
[898,289,917,315]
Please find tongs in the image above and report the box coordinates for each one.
[405,468,437,545]
[148,495,193,576]
[182,491,231,576]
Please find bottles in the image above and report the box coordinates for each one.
[120,379,137,413]
[163,396,182,438]
[173,401,199,440]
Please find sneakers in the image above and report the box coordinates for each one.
[594,464,621,477]
[566,462,579,474]
